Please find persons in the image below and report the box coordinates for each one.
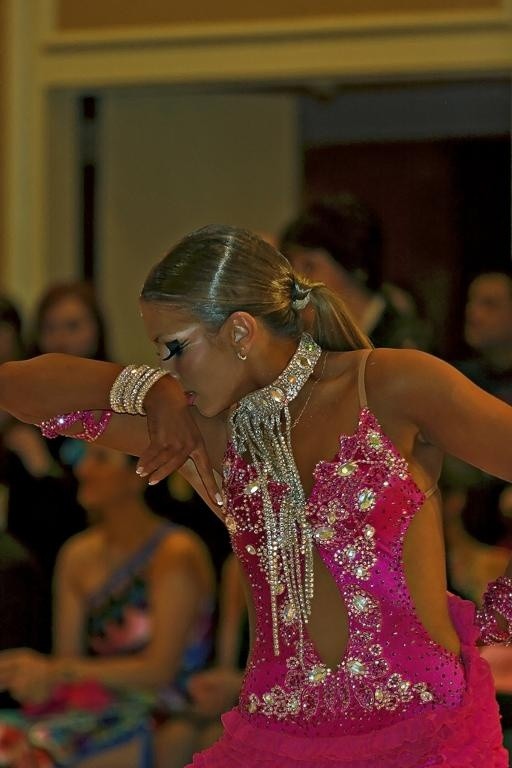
[0,224,511,768]
[440,269,511,749]
[273,191,440,357]
[3,281,252,767]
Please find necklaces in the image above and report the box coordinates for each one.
[228,334,328,670]
